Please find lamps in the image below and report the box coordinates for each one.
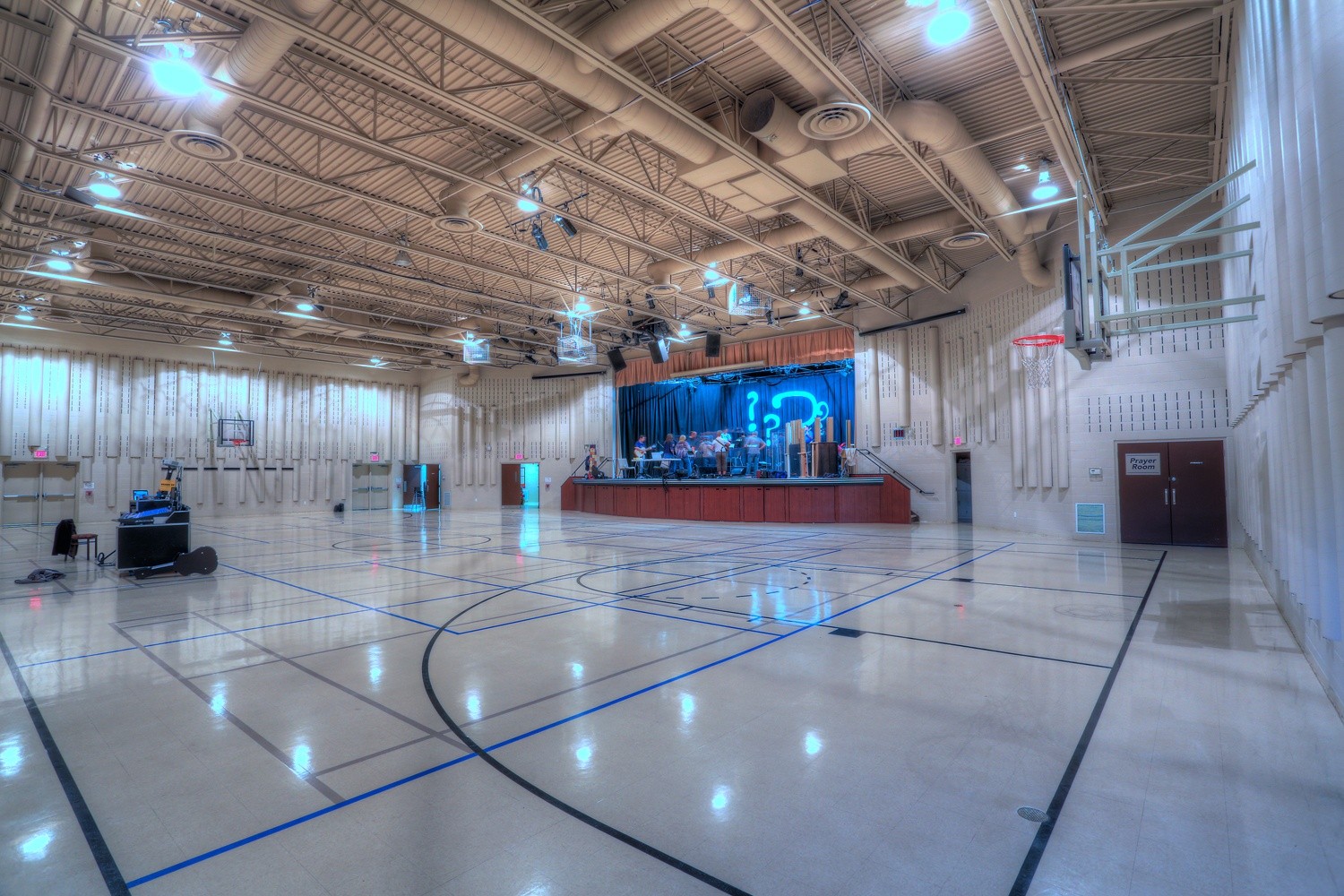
[152,38,204,96]
[90,171,121,199]
[206,179,818,368]
[1030,169,1062,201]
[14,307,37,322]
[45,248,73,272]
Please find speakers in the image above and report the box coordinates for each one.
[648,339,669,364]
[607,348,627,373]
[706,334,721,358]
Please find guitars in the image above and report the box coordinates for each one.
[134,546,218,580]
[634,444,655,458]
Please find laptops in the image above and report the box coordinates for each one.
[133,489,148,501]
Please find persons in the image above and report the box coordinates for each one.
[675,435,693,480]
[699,438,716,478]
[721,428,732,446]
[733,427,747,467]
[662,434,676,475]
[685,431,697,474]
[744,431,767,477]
[634,435,652,478]
[712,429,731,476]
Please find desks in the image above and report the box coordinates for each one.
[631,458,682,480]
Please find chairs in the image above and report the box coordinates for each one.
[61,518,98,562]
[618,458,636,479]
[651,456,672,478]
